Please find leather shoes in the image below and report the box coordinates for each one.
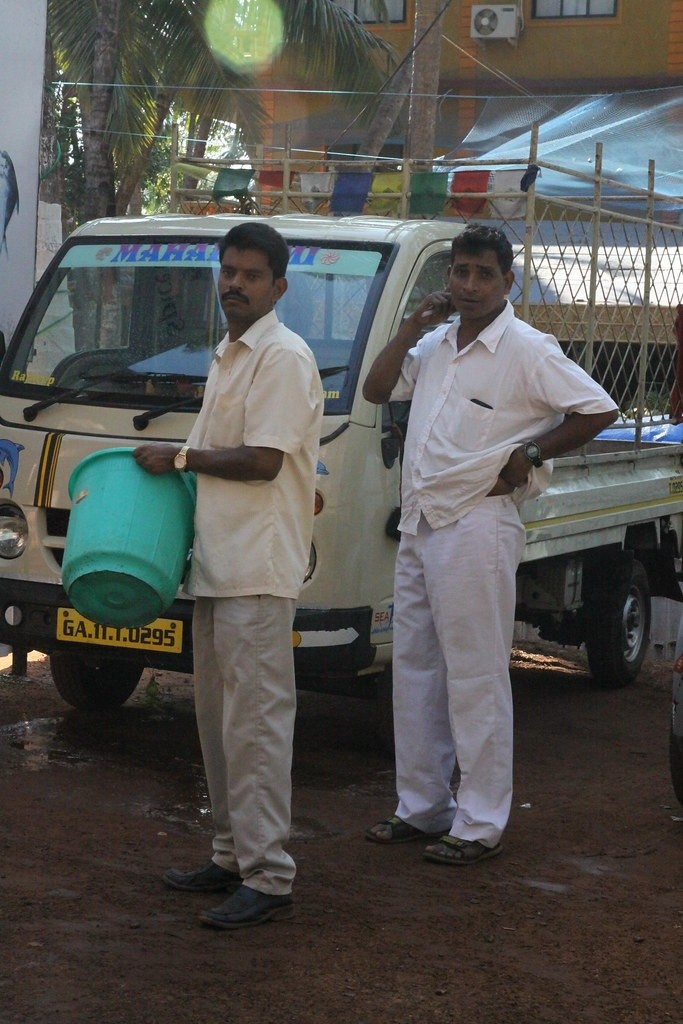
[163,862,298,928]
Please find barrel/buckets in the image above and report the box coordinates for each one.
[62,447,198,628]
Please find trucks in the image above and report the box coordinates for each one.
[0,213,683,709]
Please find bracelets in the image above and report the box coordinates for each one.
[499,469,528,487]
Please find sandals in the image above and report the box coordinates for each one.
[422,835,504,866]
[366,815,423,843]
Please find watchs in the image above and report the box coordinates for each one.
[173,447,190,472]
[524,441,543,468]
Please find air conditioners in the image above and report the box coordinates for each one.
[470,5,518,38]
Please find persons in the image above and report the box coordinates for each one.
[133,224,325,933]
[361,224,620,867]
